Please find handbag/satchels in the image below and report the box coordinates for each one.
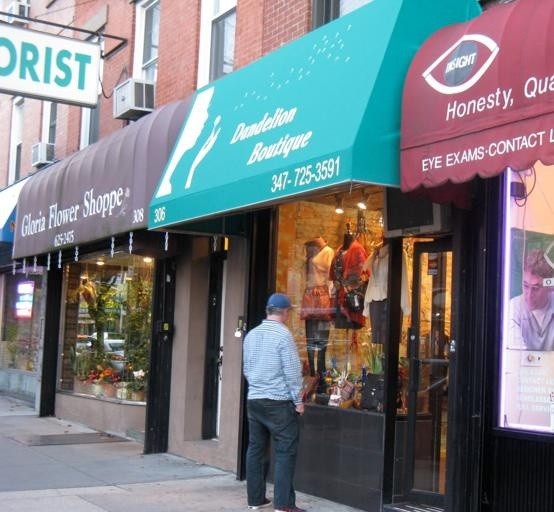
[360,372,401,410]
[344,291,364,313]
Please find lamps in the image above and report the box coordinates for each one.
[332,186,373,214]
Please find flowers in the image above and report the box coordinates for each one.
[124,368,146,391]
[78,365,117,385]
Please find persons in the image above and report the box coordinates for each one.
[239,291,307,512]
[301,230,410,414]
[510,249,554,353]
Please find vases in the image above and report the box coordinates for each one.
[91,382,105,396]
[128,387,143,401]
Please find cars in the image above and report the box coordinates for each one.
[72,327,126,378]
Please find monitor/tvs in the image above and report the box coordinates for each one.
[383,185,451,239]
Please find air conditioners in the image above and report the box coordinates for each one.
[112,78,155,122]
[5,1,29,26]
[27,139,52,167]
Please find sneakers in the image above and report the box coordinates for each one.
[247,498,272,509]
[275,506,308,512]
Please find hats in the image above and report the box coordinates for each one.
[266,293,300,308]
[304,235,328,249]
